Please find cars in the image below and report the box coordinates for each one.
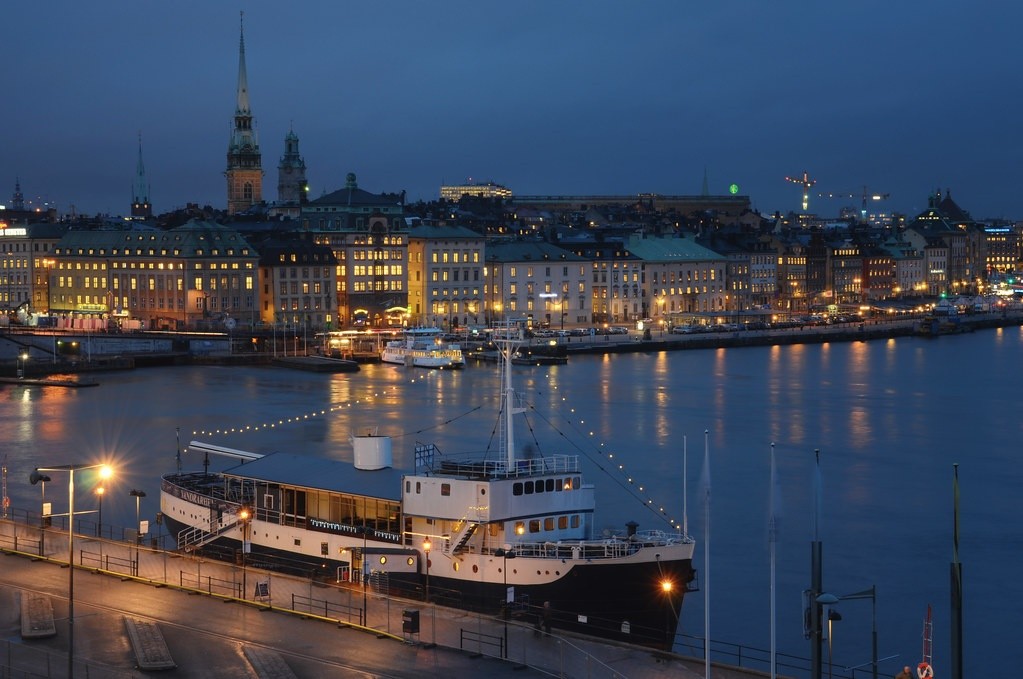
[673,314,865,335]
[441,333,485,341]
[533,326,628,338]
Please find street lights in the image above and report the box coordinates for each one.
[22,355,27,378]
[97,488,104,537]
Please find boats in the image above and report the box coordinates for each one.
[381,316,465,369]
[161,317,696,651]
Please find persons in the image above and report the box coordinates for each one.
[896,666,913,679]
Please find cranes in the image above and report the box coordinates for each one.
[785,171,816,215]
[815,185,890,223]
